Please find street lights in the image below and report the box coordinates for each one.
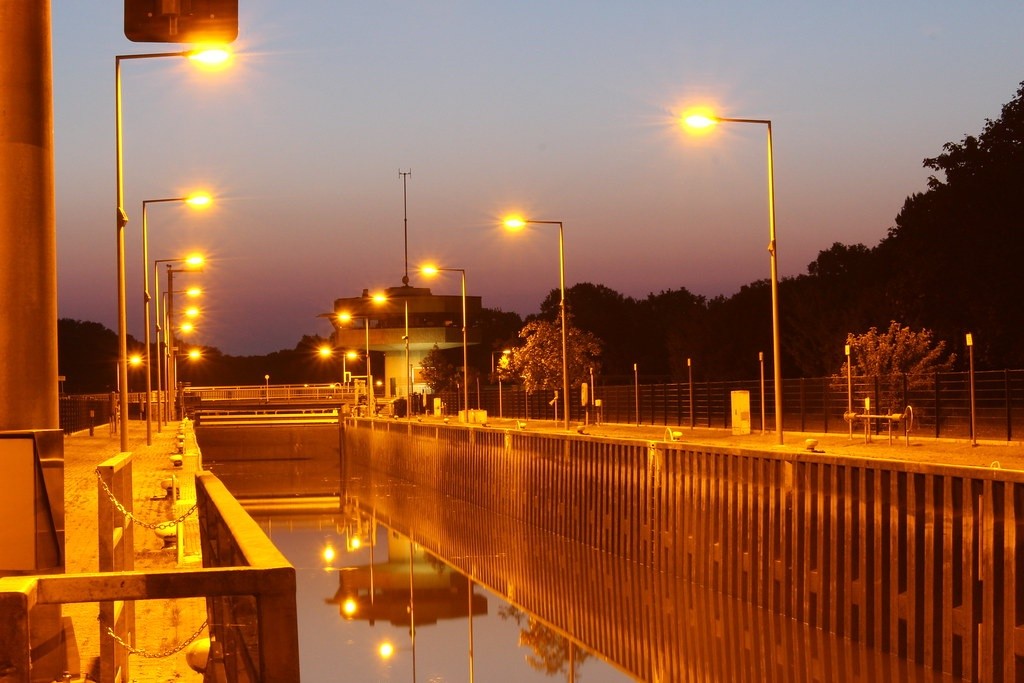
[680,104,784,447]
[380,542,415,657]
[372,292,411,420]
[419,262,469,424]
[504,215,570,431]
[116,355,141,392]
[348,349,371,377]
[113,41,235,452]
[154,253,206,434]
[141,192,211,447]
[318,346,346,388]
[163,287,200,425]
[343,516,376,625]
[338,311,371,418]
